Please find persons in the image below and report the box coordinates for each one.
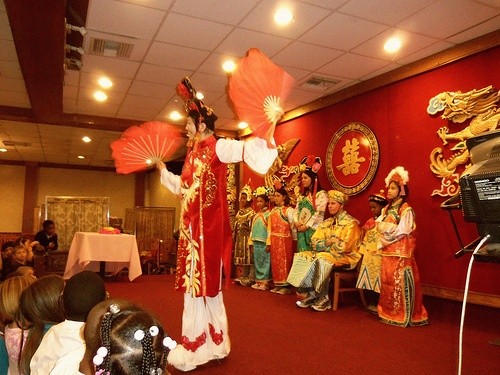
[0,266,38,375]
[288,190,361,311]
[356,190,389,314]
[155,76,283,371]
[248,194,273,290]
[293,156,330,295]
[84,304,177,375]
[232,184,255,285]
[20,275,67,375]
[375,166,428,326]
[35,220,58,251]
[30,271,107,375]
[0,236,46,282]
[48,300,144,375]
[265,189,298,294]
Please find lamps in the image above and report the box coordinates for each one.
[66,24,88,38]
[64,58,82,68]
[65,44,85,55]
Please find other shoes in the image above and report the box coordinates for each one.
[277,288,291,295]
[251,283,262,290]
[259,282,270,291]
[270,286,282,293]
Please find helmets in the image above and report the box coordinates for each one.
[238,178,253,202]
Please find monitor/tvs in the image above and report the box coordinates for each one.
[459,129,500,243]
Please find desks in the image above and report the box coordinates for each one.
[64,231,143,282]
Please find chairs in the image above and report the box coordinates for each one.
[139,239,162,275]
[47,248,68,277]
[330,256,367,310]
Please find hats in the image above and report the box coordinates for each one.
[369,190,387,206]
[328,190,347,204]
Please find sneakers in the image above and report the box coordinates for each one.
[296,294,317,308]
[311,297,331,311]
